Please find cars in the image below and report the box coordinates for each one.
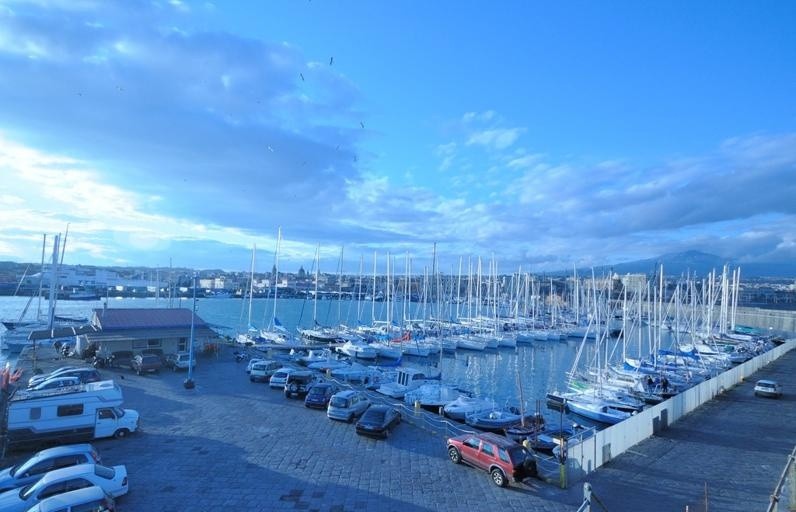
[26,365,104,391]
[0,443,129,512]
[304,383,401,439]
[246,358,297,390]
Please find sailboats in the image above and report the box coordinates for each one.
[546,261,791,424]
[0,222,101,353]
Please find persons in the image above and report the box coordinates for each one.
[60,341,70,355]
[131,352,143,370]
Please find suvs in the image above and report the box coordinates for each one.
[446,432,537,488]
[284,370,325,399]
[130,351,197,376]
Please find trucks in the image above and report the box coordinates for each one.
[5,379,140,453]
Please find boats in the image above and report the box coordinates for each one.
[295,355,579,449]
